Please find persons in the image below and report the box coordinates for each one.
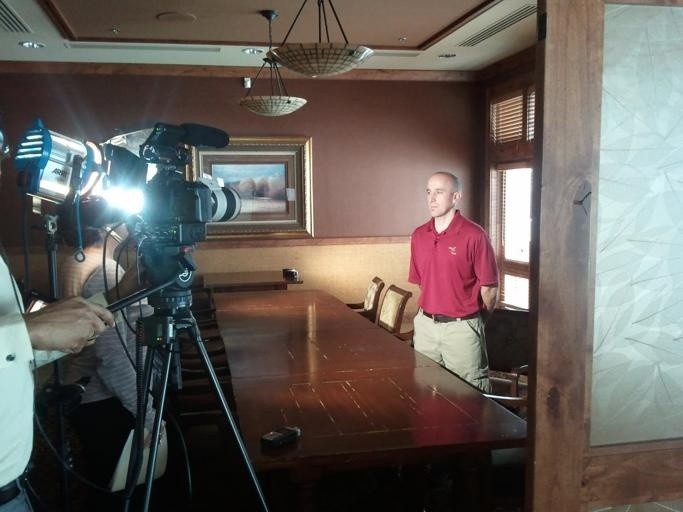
[412,349,485,448]
[0,253,150,512]
[404,171,501,396]
[51,202,164,510]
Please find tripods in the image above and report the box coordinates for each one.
[98,243,272,512]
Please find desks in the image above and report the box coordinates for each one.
[211,287,531,512]
[201,271,303,296]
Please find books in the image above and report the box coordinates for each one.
[108,423,169,494]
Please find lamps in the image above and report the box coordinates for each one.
[266,0,373,78]
[239,10,307,117]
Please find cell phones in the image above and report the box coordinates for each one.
[261,426,301,447]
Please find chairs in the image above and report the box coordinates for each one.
[345,276,530,421]
[168,284,237,414]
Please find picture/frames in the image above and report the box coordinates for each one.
[185,134,315,240]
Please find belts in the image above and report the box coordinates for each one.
[422,310,480,323]
[0,468,28,506]
